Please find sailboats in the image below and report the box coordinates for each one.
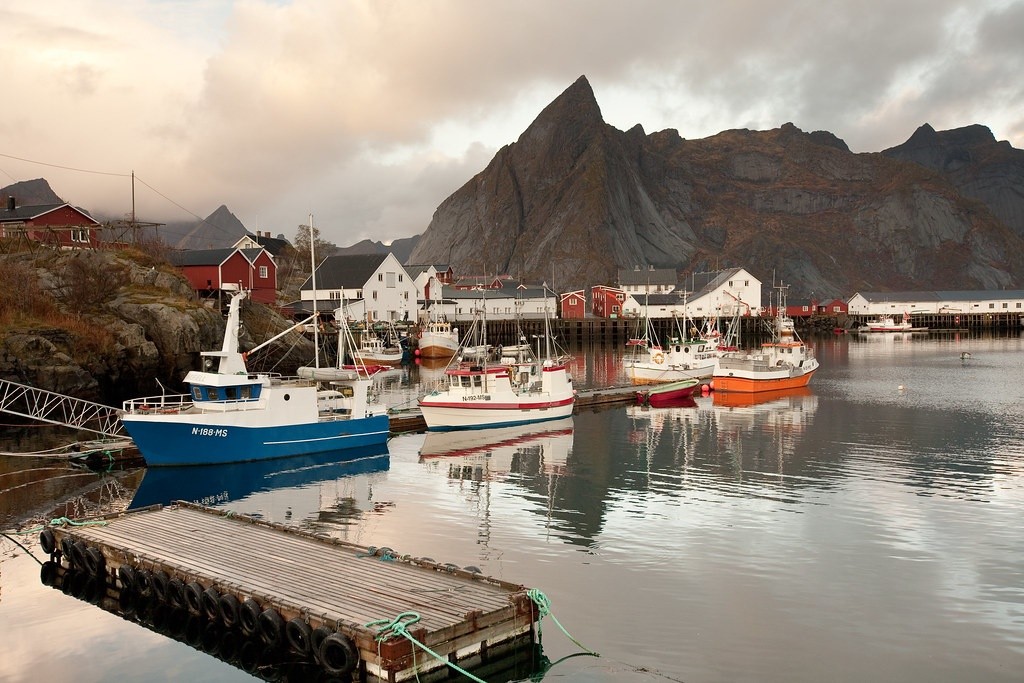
[247,210,820,432]
[416,416,574,548]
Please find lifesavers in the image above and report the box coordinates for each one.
[654,354,664,364]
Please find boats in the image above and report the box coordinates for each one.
[124,442,394,514]
[866,313,913,332]
[114,278,390,469]
